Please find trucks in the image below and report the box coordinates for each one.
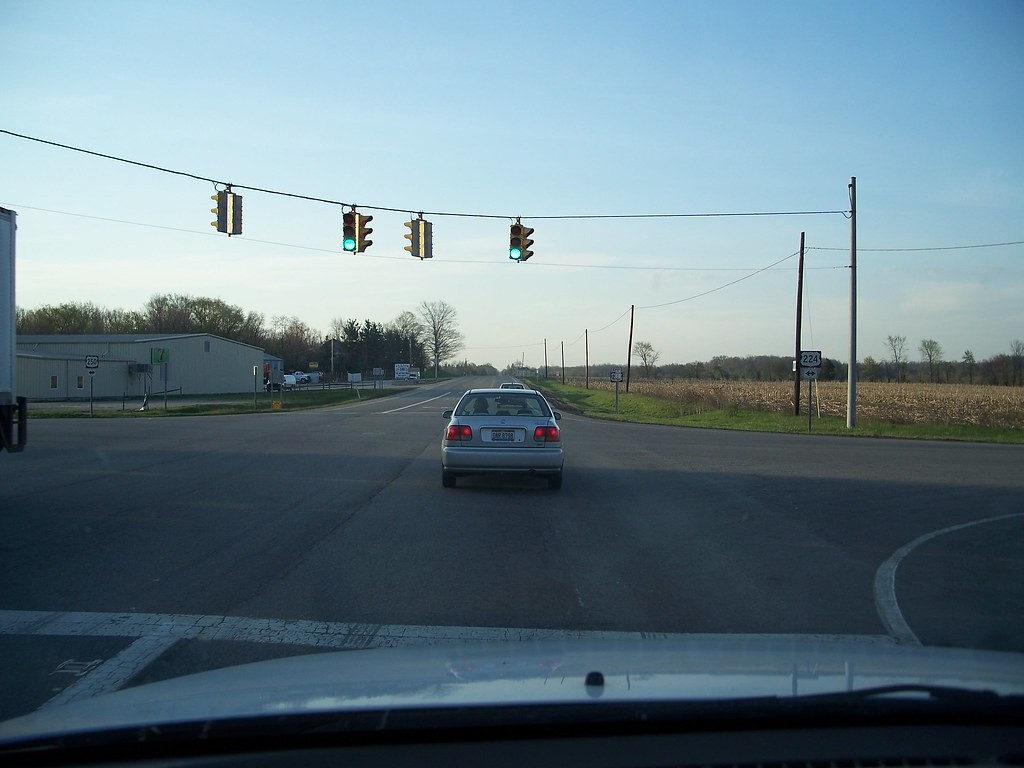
[294,371,311,384]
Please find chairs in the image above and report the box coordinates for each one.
[496,410,510,415]
[518,410,533,414]
[473,409,488,414]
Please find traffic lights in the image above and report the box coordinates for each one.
[404,219,419,257]
[343,212,356,253]
[211,191,228,233]
[356,213,374,252]
[521,226,535,262]
[509,225,523,260]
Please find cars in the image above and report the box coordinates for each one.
[498,382,528,407]
[439,388,566,491]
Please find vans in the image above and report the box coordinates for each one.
[409,372,420,381]
[266,375,297,392]
